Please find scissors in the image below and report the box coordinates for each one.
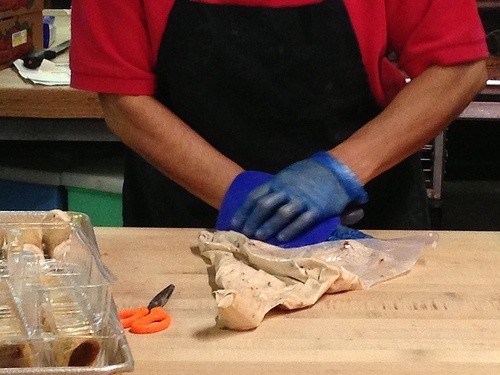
[117,283,176,335]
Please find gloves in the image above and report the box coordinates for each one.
[230,150,369,243]
[216,169,372,248]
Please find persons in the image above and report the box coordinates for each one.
[70,0,488,244]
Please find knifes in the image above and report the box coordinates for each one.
[23,40,71,70]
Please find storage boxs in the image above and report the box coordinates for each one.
[0,0,43,71]
[0,158,125,227]
